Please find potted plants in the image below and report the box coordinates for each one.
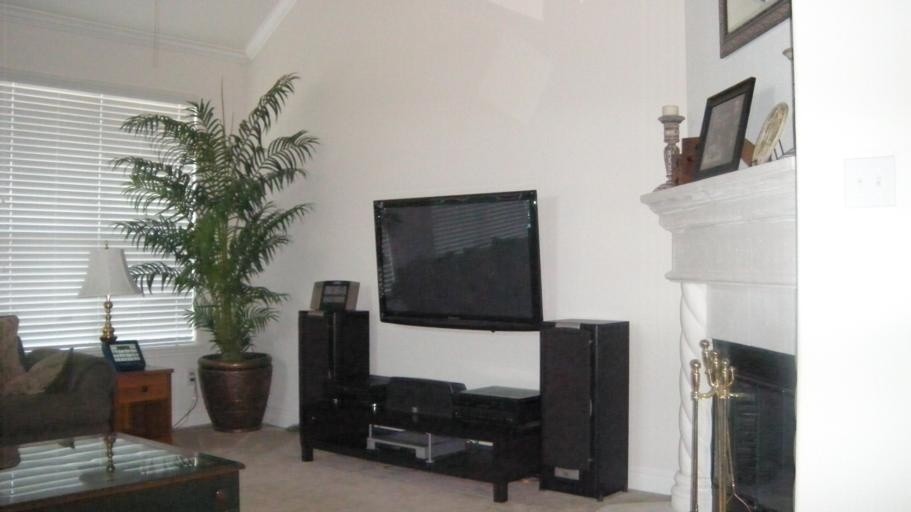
[107,72,322,433]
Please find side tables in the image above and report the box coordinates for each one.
[113,365,175,438]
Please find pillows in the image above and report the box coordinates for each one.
[2,315,74,398]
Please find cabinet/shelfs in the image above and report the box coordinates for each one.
[302,374,541,501]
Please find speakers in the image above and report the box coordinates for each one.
[539,317,630,501]
[297,310,370,437]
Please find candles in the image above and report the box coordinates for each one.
[662,105,680,116]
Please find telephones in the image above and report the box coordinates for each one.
[102,340,145,372]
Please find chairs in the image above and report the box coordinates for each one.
[2,347,110,448]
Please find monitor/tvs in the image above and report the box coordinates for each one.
[374,189,543,332]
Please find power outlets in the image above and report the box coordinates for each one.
[187,369,198,385]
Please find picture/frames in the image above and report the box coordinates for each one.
[683,77,789,185]
[720,0,793,59]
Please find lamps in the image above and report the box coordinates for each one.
[79,246,141,342]
